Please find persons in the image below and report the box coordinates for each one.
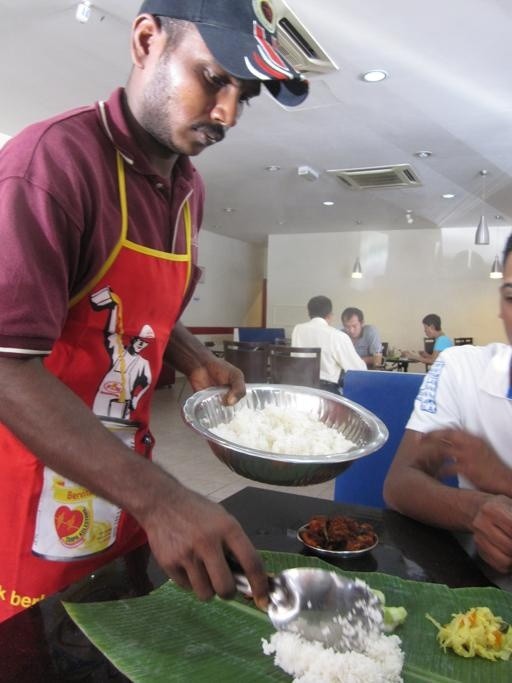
[2,0,343,639]
[338,306,383,372]
[378,234,511,584]
[397,312,454,368]
[91,302,157,421]
[288,294,369,395]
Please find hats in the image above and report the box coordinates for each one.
[138,0,309,106]
[138,326,156,343]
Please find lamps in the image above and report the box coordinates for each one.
[474,169,503,279]
[350,257,362,278]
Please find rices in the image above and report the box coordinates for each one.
[260,617,406,683]
[208,402,357,456]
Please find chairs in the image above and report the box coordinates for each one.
[333,368,460,513]
[222,338,321,389]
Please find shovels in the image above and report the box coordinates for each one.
[215,556,385,654]
